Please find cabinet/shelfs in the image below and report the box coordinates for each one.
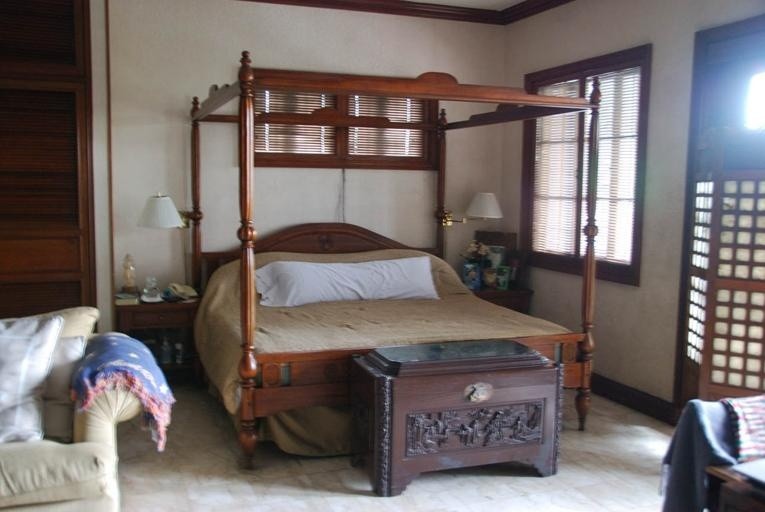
[348,340,566,494]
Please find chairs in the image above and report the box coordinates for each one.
[0,306,148,512]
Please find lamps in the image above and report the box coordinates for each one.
[135,191,187,305]
[468,190,504,232]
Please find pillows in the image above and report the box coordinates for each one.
[252,256,443,308]
[2,316,62,442]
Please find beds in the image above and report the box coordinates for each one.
[193,221,596,469]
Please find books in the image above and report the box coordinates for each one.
[115,292,142,306]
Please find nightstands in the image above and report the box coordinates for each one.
[112,301,200,386]
[476,285,537,315]
